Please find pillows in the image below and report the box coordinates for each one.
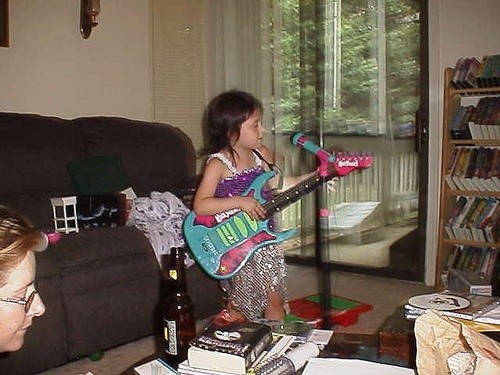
[68,154,132,195]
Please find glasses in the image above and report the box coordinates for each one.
[0,279,40,313]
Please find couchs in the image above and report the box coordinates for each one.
[0,112,228,375]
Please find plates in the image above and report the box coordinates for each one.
[408,294,471,311]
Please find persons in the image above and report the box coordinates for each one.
[0,204,49,352]
[190,90,338,323]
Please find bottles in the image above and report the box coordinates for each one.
[162,247,195,366]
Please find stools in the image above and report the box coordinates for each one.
[329,199,384,248]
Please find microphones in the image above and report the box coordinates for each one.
[289,132,335,162]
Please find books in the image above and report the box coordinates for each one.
[445,147,500,192]
[443,195,500,250]
[177,314,306,375]
[451,53,500,96]
[403,288,500,325]
[441,245,500,297]
[450,97,500,146]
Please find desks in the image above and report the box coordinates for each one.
[119,341,417,374]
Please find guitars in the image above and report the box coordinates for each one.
[182,148,375,280]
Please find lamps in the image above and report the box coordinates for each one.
[80,0,100,39]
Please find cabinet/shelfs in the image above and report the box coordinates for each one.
[434,67,500,292]
[48,195,79,235]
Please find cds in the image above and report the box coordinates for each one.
[408,293,471,311]
